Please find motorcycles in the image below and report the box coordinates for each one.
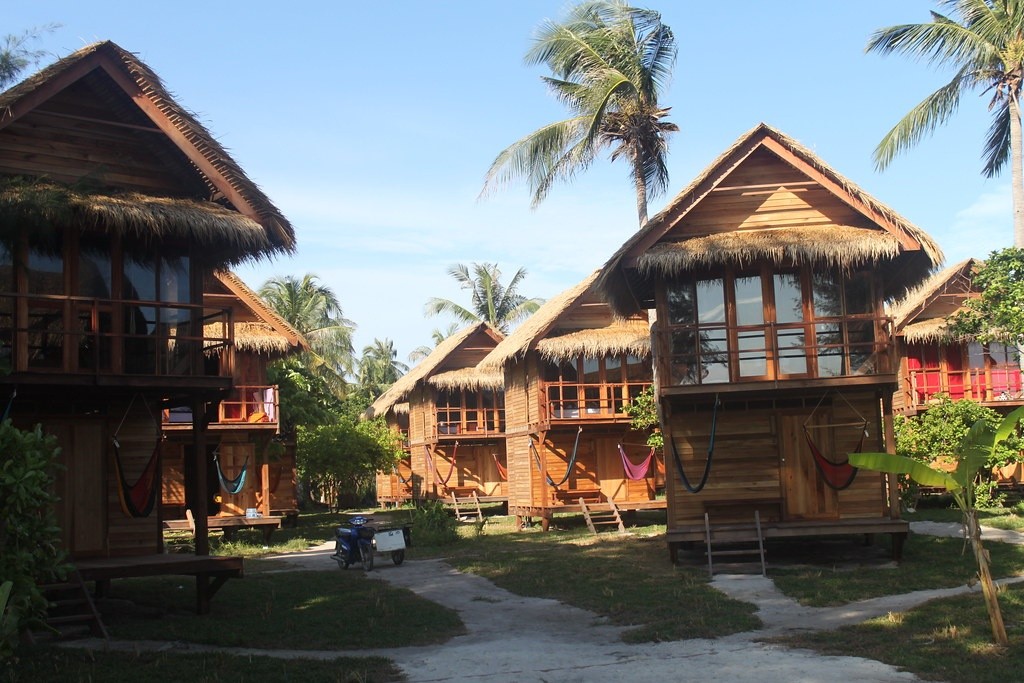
[330,517,414,571]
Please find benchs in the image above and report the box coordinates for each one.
[550,489,602,505]
[703,497,785,523]
[442,486,479,498]
[42,552,244,615]
